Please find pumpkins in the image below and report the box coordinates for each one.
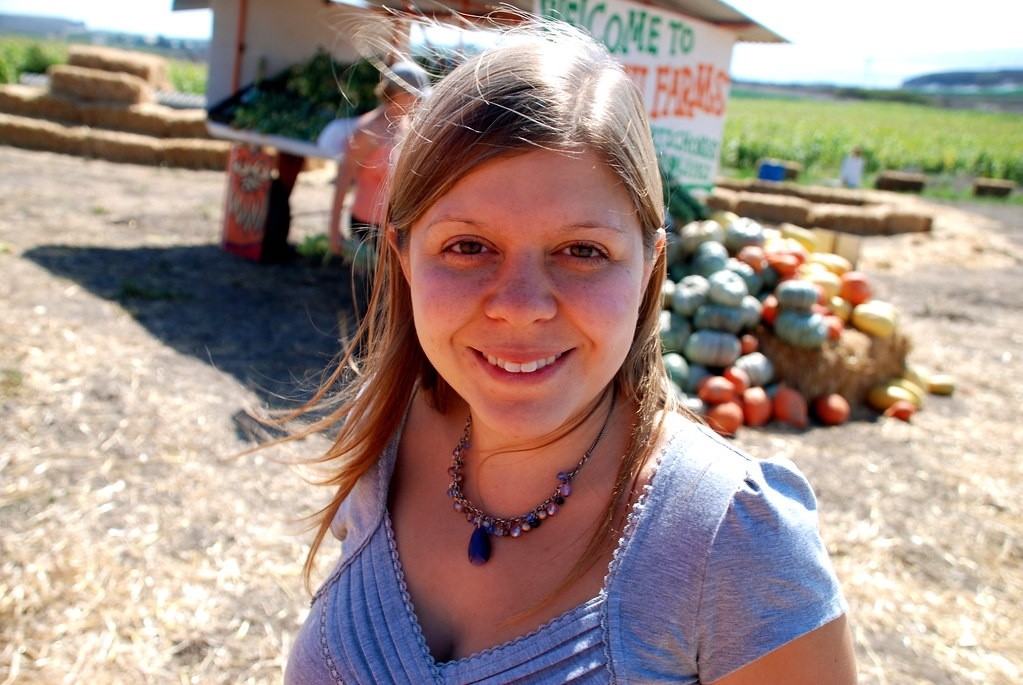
[660,212,929,437]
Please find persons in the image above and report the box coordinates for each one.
[330,62,431,356]
[841,146,864,188]
[281,20,859,685]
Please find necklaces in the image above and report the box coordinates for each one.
[447,377,618,567]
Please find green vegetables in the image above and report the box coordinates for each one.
[231,43,381,145]
